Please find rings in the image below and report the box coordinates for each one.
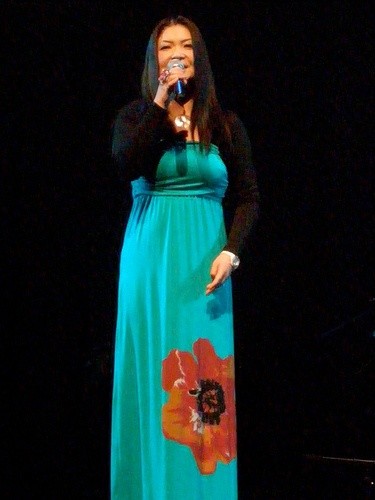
[158,70,170,84]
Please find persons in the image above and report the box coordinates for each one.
[109,16,263,500]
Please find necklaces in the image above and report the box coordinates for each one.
[170,101,192,130]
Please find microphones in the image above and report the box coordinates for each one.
[167,59,186,98]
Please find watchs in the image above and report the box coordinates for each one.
[221,251,241,270]
